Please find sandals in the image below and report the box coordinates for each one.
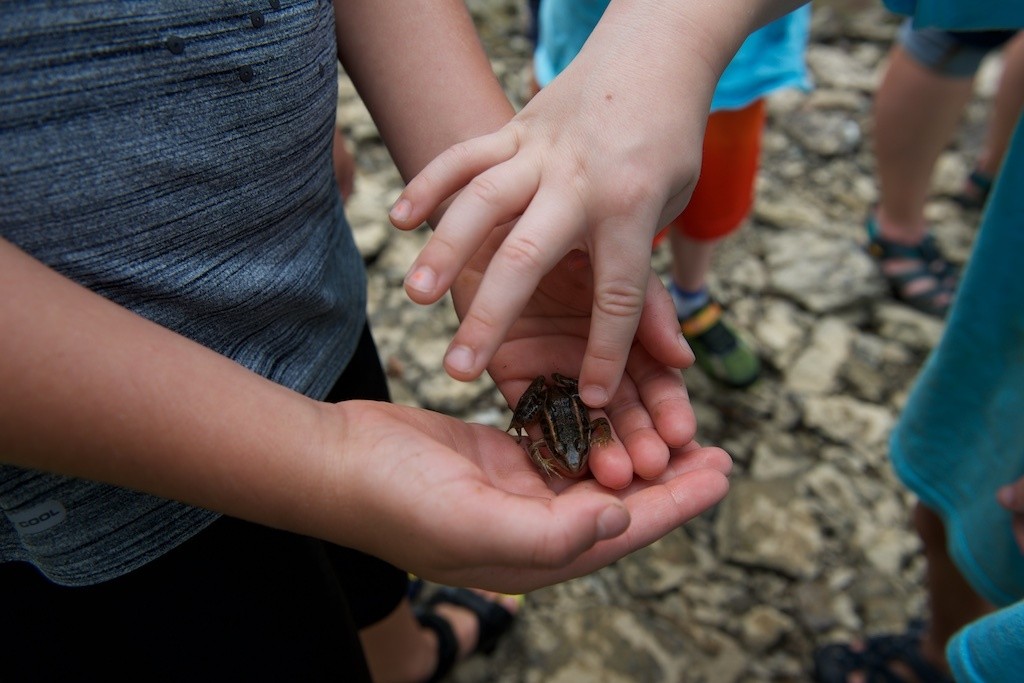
[413,586,517,677]
[865,210,960,318]
[955,171,992,219]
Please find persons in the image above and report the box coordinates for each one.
[0,0,733,682]
[387,0,1024,683]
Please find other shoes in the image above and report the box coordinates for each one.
[679,298,759,388]
[810,628,952,683]
